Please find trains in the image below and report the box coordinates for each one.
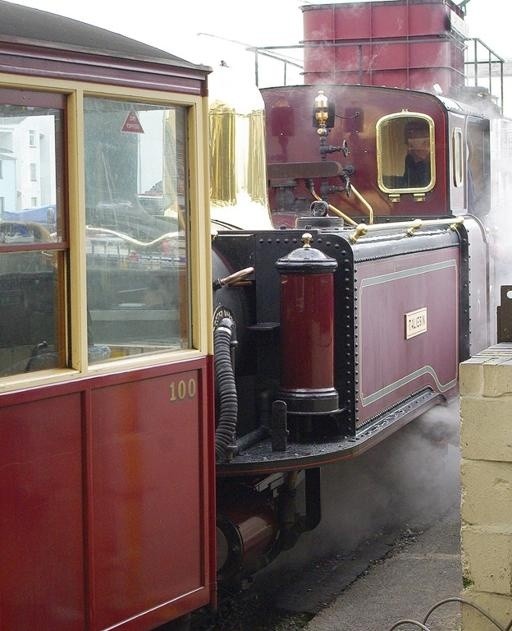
[0,0,511,631]
